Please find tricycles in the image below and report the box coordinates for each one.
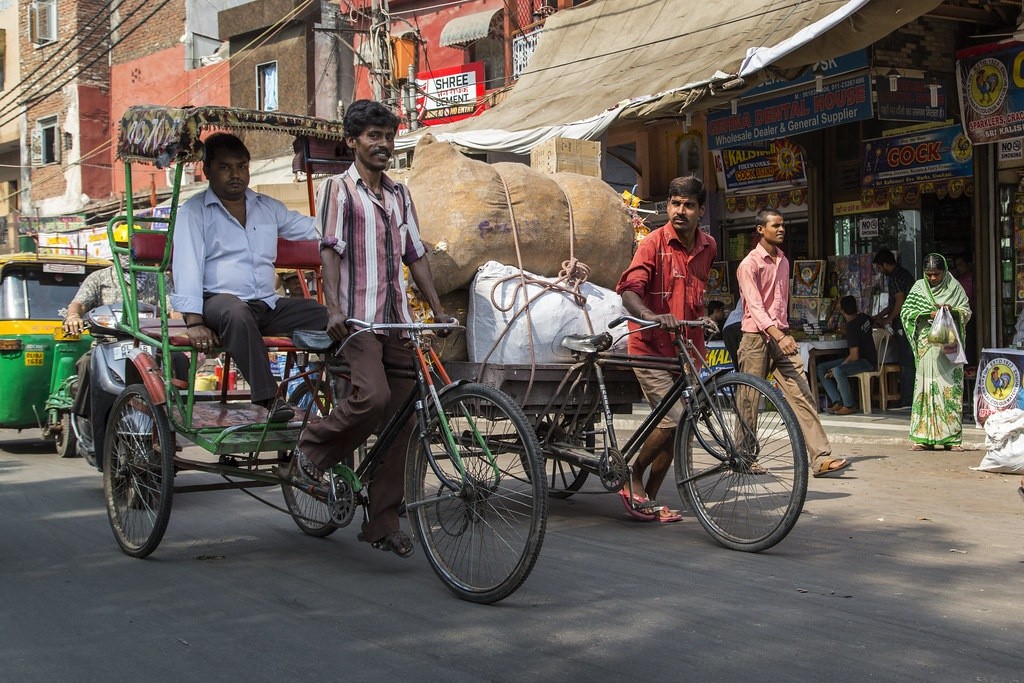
[356,315,808,553]
[102,105,549,603]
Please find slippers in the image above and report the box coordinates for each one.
[650,500,682,523]
[732,462,765,475]
[945,444,962,452]
[812,458,850,478]
[910,441,934,451]
[615,475,654,519]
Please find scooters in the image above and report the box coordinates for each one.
[62,301,171,508]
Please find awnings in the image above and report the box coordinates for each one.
[438,8,503,50]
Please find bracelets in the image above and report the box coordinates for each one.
[778,334,786,343]
[68,313,81,319]
[641,310,653,318]
[187,322,204,329]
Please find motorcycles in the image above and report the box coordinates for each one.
[0,238,114,458]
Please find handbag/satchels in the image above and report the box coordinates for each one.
[928,307,968,365]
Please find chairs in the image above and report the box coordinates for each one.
[825,329,890,414]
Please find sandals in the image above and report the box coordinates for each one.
[363,520,414,558]
[298,430,325,485]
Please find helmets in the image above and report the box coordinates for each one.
[114,222,142,243]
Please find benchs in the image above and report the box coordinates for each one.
[130,229,329,434]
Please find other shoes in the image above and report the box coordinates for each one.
[265,399,295,422]
[835,404,858,415]
[823,402,842,413]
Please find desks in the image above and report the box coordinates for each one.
[698,341,848,411]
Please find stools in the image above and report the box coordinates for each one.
[857,364,901,411]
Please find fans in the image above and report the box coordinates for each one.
[965,0,1024,45]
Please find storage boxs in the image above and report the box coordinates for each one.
[529,136,602,180]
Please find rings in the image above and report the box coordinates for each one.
[196,343,201,345]
[209,342,213,343]
[202,343,207,345]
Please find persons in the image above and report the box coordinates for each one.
[278,277,320,415]
[63,224,191,455]
[955,255,975,368]
[722,297,743,375]
[616,176,717,523]
[817,295,877,416]
[704,300,727,341]
[901,253,972,450]
[734,208,852,477]
[296,99,459,558]
[171,133,322,424]
[823,272,837,297]
[872,249,917,410]
